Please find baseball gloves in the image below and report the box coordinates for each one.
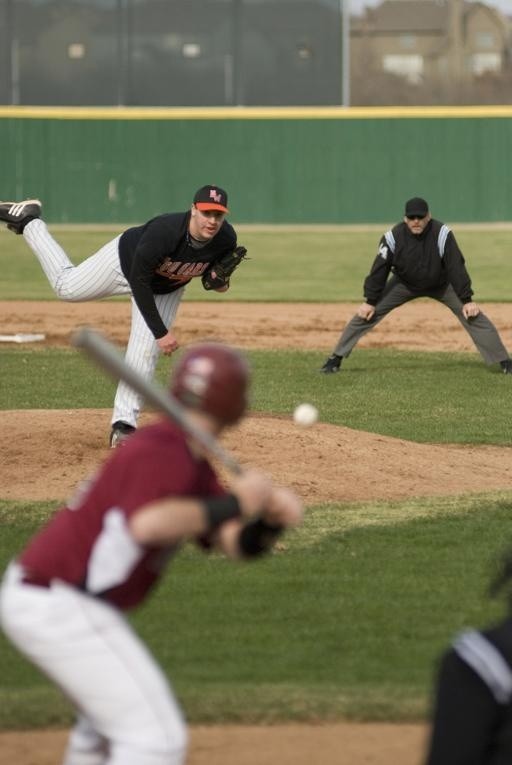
[201,247,252,291]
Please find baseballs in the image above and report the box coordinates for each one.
[294,404,319,425]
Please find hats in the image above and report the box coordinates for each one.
[192,183,230,217]
[404,196,429,218]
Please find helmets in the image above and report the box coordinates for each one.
[169,341,251,426]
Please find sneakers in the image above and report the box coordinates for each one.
[0,199,42,235]
[500,359,512,373]
[318,356,342,373]
[107,421,136,448]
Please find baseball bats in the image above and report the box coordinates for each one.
[70,329,274,508]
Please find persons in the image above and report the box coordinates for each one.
[4,342,307,765]
[318,196,511,378]
[418,554,511,765]
[0,179,243,445]
